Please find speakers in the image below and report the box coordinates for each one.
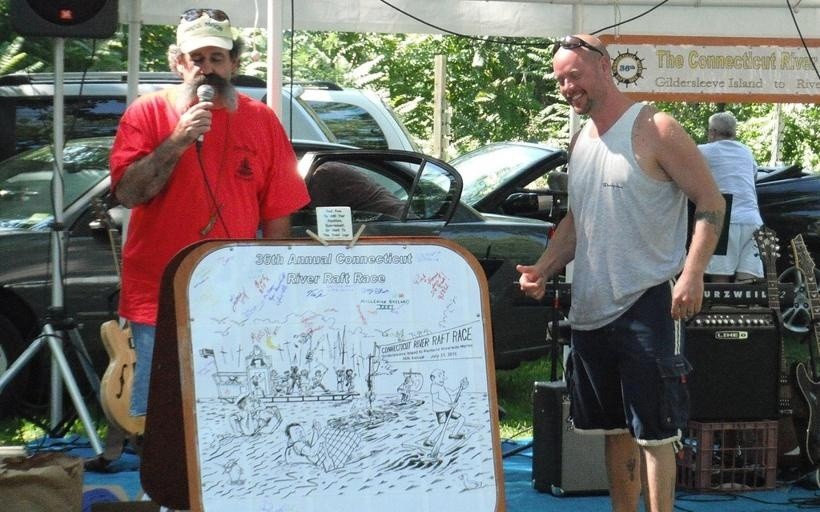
[10,0,117,37]
[679,306,782,422]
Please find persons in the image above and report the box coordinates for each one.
[110,9,311,417]
[308,157,414,220]
[697,111,766,283]
[517,34,727,512]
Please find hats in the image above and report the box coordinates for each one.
[176,18,233,53]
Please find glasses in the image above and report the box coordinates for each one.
[550,36,605,57]
[180,9,228,21]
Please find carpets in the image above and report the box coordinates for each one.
[29,433,820,511]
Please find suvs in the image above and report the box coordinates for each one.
[278,80,424,156]
[0,69,340,162]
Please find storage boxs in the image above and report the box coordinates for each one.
[0,446,83,512]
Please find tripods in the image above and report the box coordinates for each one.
[0,37,131,468]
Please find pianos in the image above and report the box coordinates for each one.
[675,278,793,332]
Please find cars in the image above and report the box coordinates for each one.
[0,136,559,412]
[420,139,820,339]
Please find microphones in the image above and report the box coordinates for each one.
[196,84,215,148]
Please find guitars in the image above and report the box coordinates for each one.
[90,195,146,440]
[752,224,797,454]
[786,230,820,464]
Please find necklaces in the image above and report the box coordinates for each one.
[199,110,232,236]
